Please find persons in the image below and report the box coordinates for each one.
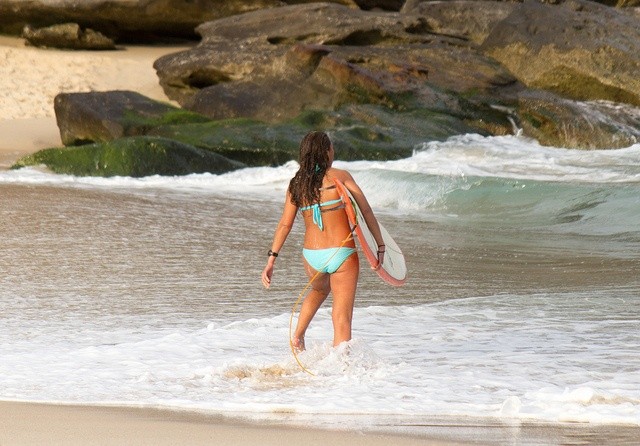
[260,131,385,352]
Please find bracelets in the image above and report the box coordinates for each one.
[376,250,385,255]
[268,250,278,257]
[378,245,385,248]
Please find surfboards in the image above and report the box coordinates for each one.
[318,176,408,287]
